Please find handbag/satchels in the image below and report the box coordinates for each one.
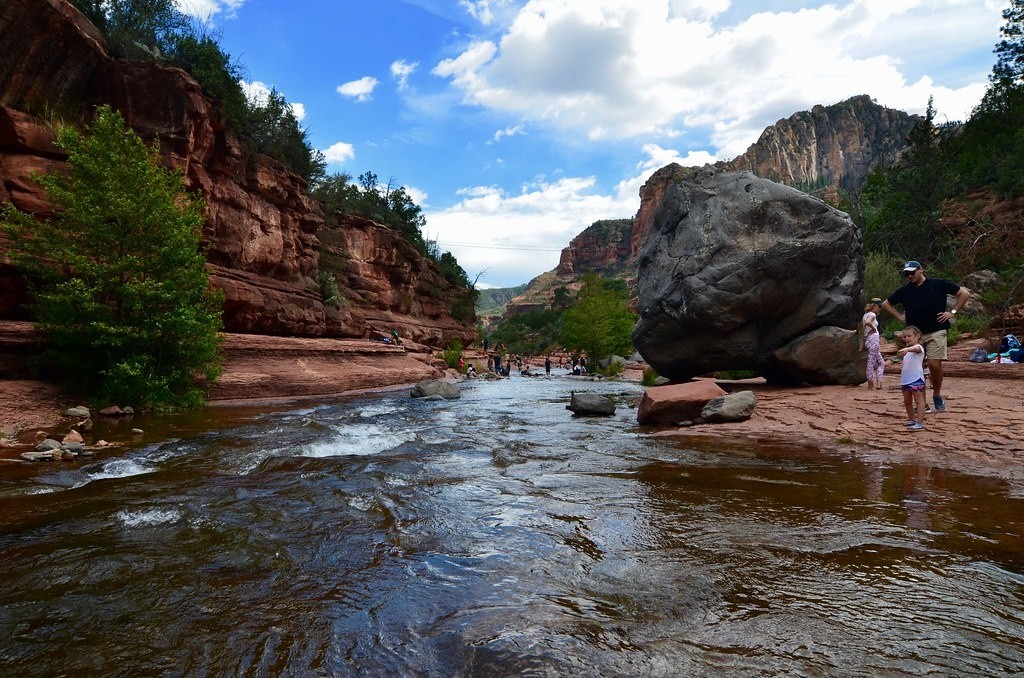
[858,338,867,352]
[1010,342,1024,363]
[969,348,987,362]
[1000,335,1021,352]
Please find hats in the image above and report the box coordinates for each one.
[902,260,922,273]
[869,298,884,307]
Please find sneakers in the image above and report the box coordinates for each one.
[911,403,931,414]
[932,396,946,412]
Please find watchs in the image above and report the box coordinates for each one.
[949,309,959,318]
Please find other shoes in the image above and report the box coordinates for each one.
[867,381,874,391]
[906,422,924,430]
[903,419,917,426]
[876,381,882,390]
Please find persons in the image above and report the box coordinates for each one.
[883,260,970,414]
[898,326,927,431]
[390,326,401,344]
[455,337,591,378]
[856,297,886,391]
